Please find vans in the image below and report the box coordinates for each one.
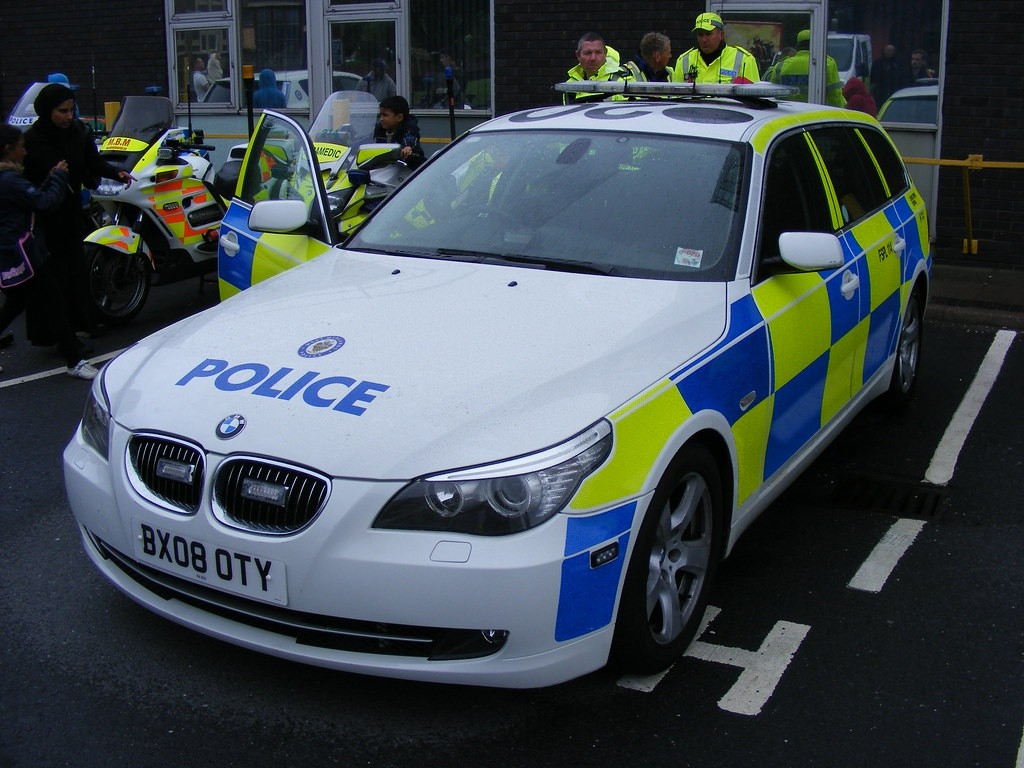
[819,35,874,87]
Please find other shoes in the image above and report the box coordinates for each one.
[61,334,94,355]
[0,334,14,349]
[69,313,108,339]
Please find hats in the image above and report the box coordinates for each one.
[690,12,724,31]
[796,29,811,45]
[34,83,76,124]
[371,59,388,71]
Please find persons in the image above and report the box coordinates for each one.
[771,30,845,109]
[842,44,935,119]
[23,84,138,337]
[375,96,427,171]
[563,32,641,106]
[356,58,395,103]
[0,124,99,380]
[620,32,674,99]
[674,13,760,84]
[439,48,472,110]
[191,49,223,102]
[253,69,286,108]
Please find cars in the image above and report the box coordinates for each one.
[63,82,931,685]
[200,69,363,109]
[874,86,941,121]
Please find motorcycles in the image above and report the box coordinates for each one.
[289,90,415,242]
[0,73,298,322]
